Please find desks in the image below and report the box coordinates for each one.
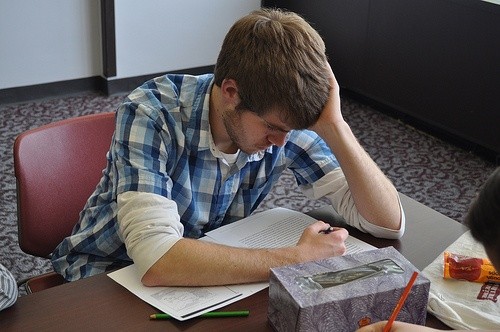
[0,193,500,332]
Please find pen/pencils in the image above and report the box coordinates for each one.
[382,271,419,332]
[150,311,250,319]
[325,227,333,233]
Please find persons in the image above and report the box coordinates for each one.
[354,168,500,332]
[52,8,406,287]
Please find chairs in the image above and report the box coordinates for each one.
[13,112,115,294]
